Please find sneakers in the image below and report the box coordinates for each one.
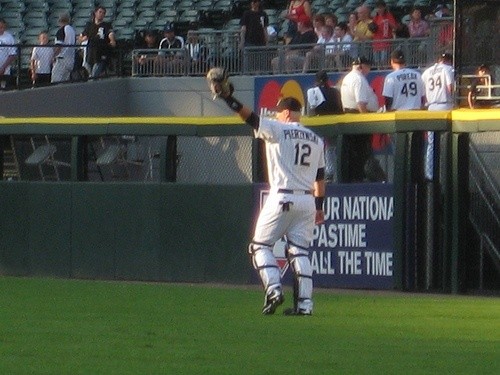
[261,287,287,314]
[297,306,313,316]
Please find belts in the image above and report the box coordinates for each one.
[278,189,313,194]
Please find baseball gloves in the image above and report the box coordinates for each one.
[206,66,234,101]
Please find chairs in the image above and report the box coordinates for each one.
[0,0,454,70]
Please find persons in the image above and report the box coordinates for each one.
[381,49,427,111]
[304,71,343,184]
[219,89,327,316]
[340,56,380,183]
[467,63,496,110]
[0,0,454,89]
[421,52,455,181]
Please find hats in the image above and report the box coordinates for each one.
[390,50,406,59]
[441,52,453,58]
[352,55,372,66]
[274,96,301,112]
